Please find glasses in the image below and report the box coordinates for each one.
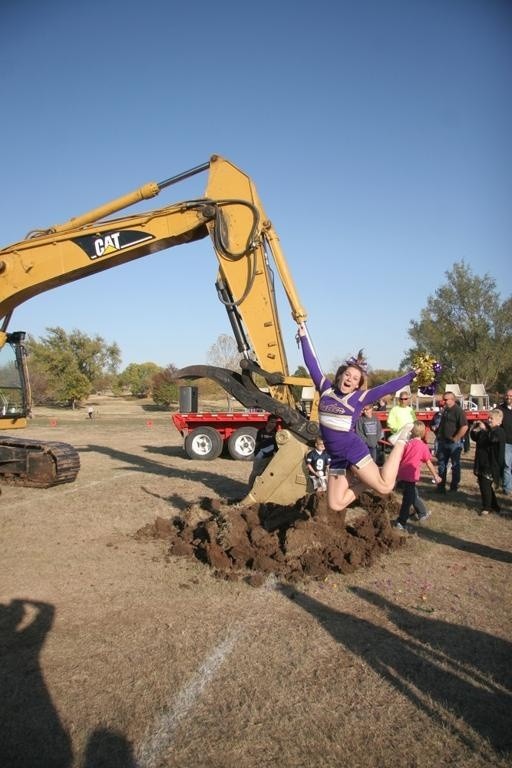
[400,397,408,400]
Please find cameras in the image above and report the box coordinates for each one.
[475,423,479,428]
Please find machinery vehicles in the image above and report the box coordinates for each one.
[1,154,336,530]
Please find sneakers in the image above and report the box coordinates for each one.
[393,522,408,530]
[420,510,431,521]
[481,510,490,515]
[389,423,414,445]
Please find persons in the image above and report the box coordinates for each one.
[290,322,424,512]
[385,389,420,441]
[86,405,95,421]
[391,420,443,530]
[305,438,333,495]
[496,387,512,496]
[435,391,470,495]
[468,406,506,517]
[359,402,384,467]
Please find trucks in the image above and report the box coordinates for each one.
[168,405,502,464]
